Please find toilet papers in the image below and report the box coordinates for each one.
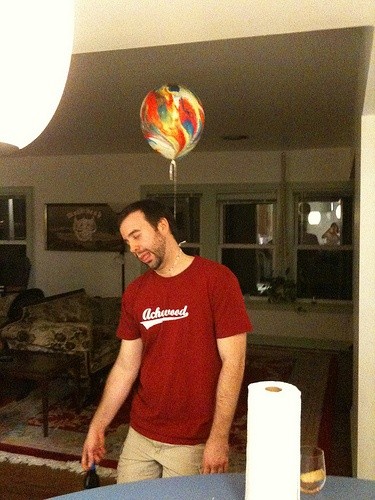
[245,380,302,500]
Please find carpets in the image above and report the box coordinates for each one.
[0,335,338,479]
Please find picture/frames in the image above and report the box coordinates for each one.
[42,203,128,253]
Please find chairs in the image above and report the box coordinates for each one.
[0,289,44,325]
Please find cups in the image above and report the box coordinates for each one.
[300,445,326,495]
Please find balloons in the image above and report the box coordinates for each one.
[139,84,205,159]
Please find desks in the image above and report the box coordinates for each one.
[45,474,375,500]
[0,348,82,437]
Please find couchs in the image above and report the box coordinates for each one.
[0,289,121,392]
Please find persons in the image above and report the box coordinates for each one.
[81,199,253,485]
[322,222,341,245]
